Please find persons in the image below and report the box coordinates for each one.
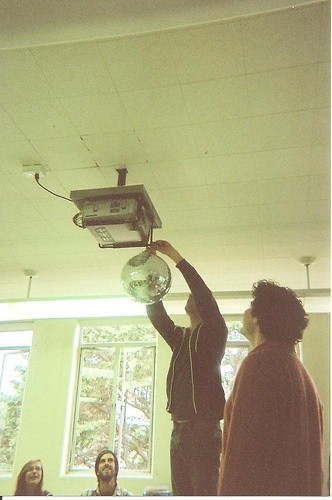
[216,278,324,496]
[80,450,133,496]
[14,458,54,496]
[145,240,228,496]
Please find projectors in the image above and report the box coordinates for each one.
[80,196,149,246]
